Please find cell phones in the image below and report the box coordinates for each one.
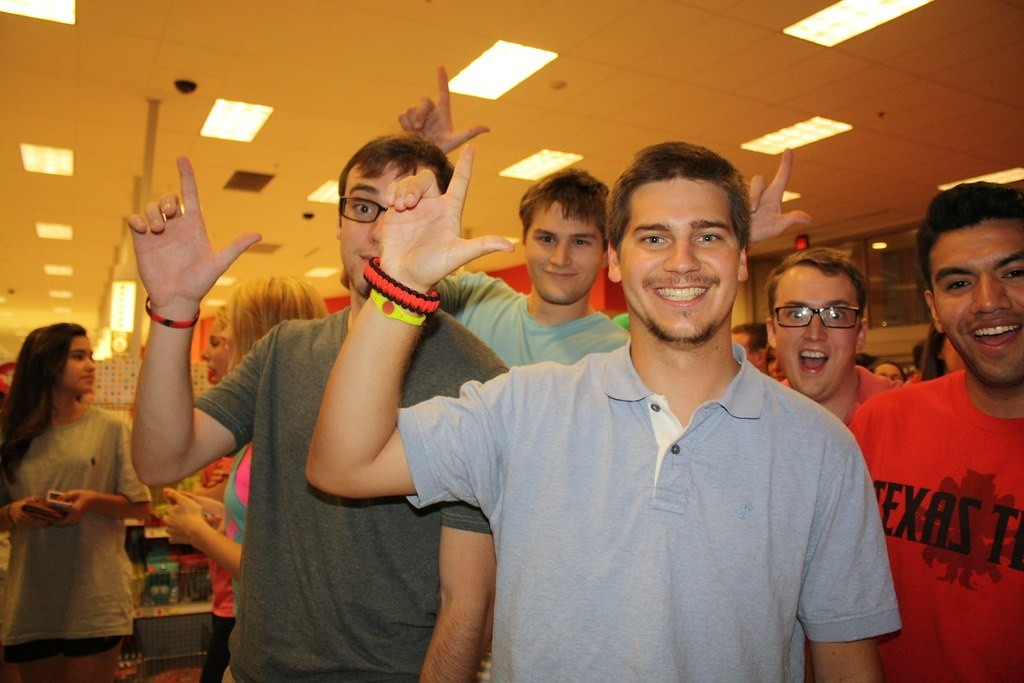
[46,490,72,509]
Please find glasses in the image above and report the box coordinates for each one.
[770,305,861,329]
[338,196,387,223]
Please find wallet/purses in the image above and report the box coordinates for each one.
[20,501,65,524]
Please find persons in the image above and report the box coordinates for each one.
[305,139,903,683]
[1,321,156,683]
[156,267,329,680]
[731,145,1024,682]
[125,131,511,683]
[395,67,630,373]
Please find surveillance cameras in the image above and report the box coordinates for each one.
[304,214,314,219]
[175,80,196,94]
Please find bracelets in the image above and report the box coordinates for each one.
[142,294,199,330]
[362,259,440,327]
